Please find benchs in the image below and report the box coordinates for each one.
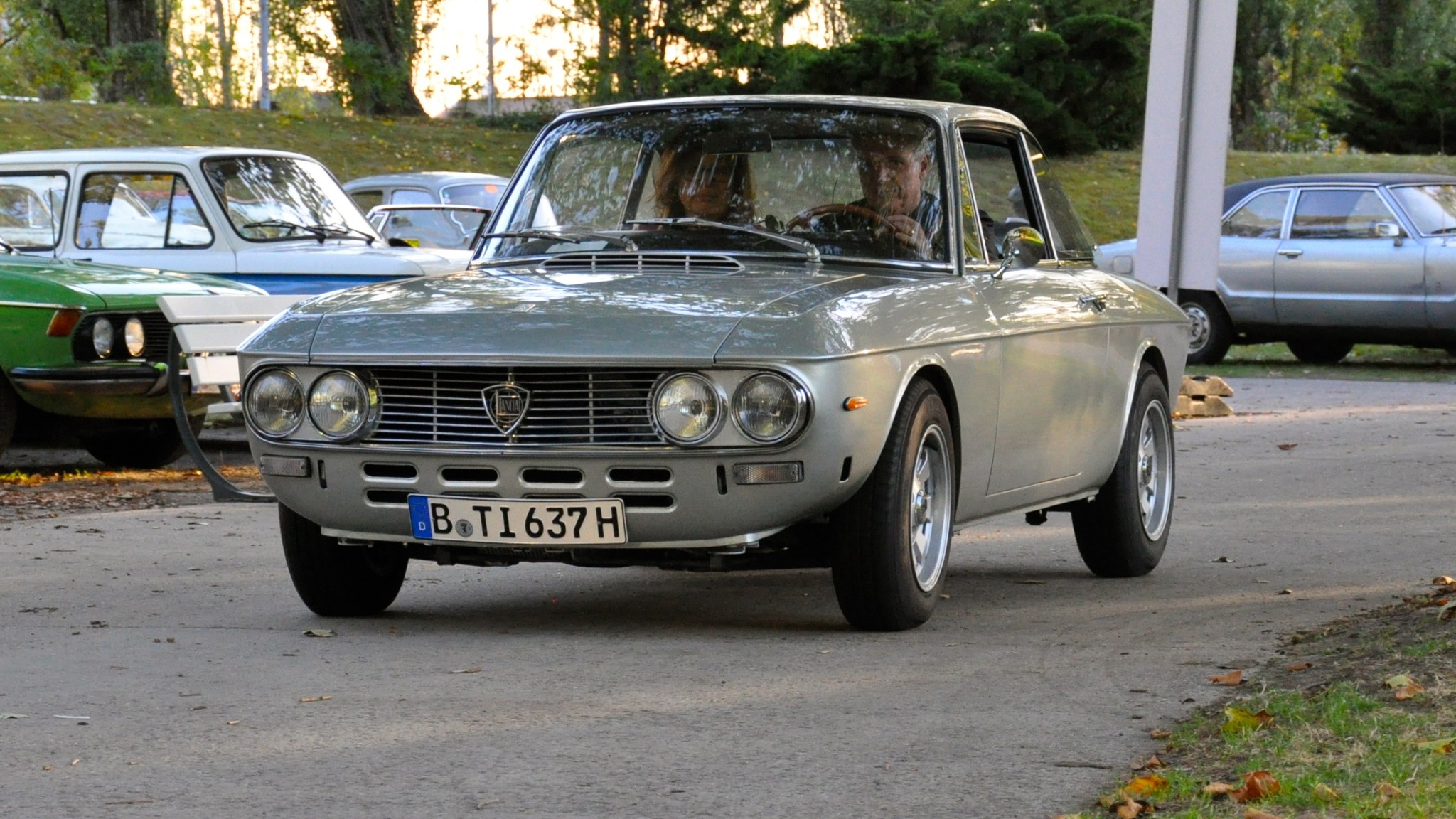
[154,295,319,503]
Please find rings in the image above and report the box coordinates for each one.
[910,230,914,236]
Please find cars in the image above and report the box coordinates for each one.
[0,240,269,470]
[237,94,1191,629]
[0,172,560,250]
[1096,174,1456,367]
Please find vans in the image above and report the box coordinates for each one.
[0,146,490,295]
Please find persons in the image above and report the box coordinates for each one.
[800,136,1000,262]
[633,144,757,230]
